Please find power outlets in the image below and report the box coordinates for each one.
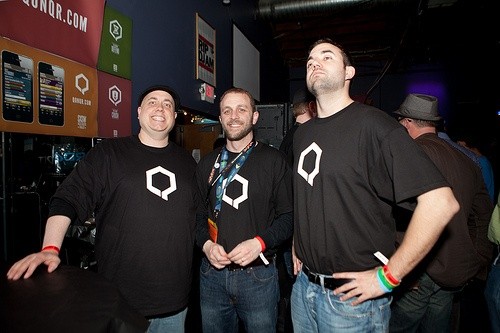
[200,83,216,104]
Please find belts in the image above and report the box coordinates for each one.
[301,263,398,300]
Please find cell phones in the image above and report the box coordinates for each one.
[1,50,35,124]
[37,61,65,127]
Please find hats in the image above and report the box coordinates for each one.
[138,84,180,109]
[393,93,443,121]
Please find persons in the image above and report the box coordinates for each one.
[7,87,198,333]
[195,88,294,333]
[279,38,500,333]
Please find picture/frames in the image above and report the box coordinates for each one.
[195,12,216,86]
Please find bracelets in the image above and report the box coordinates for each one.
[41,245,60,253]
[377,265,400,293]
[254,235,266,253]
